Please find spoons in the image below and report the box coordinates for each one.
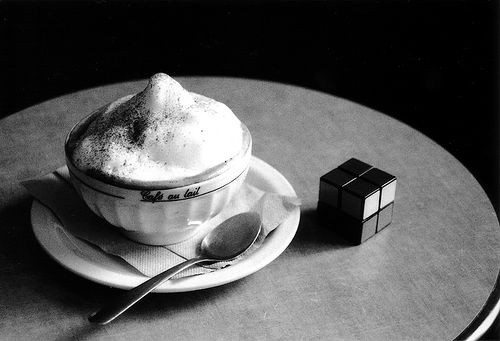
[87,211,262,326]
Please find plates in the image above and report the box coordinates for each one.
[30,165,301,293]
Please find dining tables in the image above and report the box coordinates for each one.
[0,73,499,341]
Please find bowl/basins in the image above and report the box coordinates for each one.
[65,106,252,248]
